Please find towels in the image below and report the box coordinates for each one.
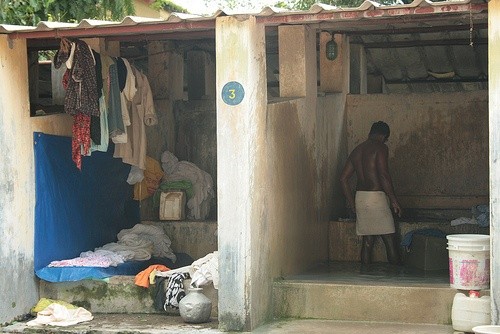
[354,190,396,236]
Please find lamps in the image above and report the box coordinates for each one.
[326,34,338,60]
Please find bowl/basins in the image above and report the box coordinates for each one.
[473,325,500,334]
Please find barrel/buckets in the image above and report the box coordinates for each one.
[446,234,489,290]
[452,291,492,333]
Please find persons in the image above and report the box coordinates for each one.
[339,120,403,278]
